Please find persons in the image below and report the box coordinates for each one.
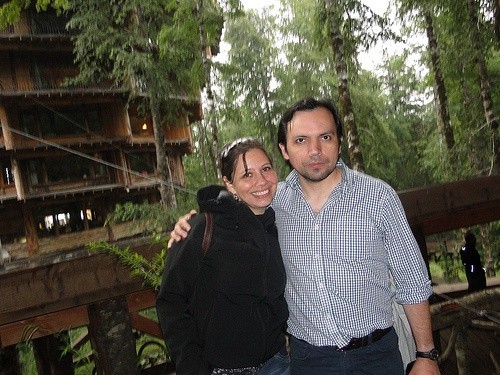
[165,96,440,375]
[460,232,487,292]
[155,131,290,375]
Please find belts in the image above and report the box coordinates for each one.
[325,326,393,350]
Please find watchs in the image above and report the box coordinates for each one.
[415,348,439,361]
